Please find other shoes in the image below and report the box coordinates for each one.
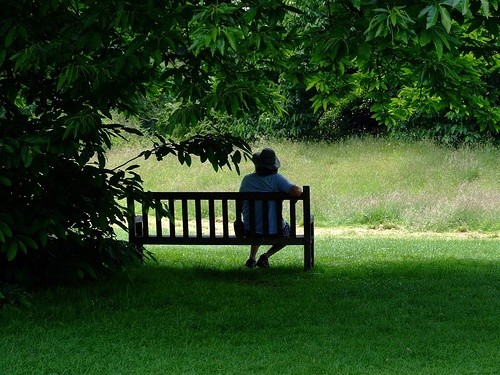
[245,257,256,268]
[257,255,269,269]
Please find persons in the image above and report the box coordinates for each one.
[234,148,301,267]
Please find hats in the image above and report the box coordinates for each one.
[252,148,280,170]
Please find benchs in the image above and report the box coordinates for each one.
[125,186,314,270]
